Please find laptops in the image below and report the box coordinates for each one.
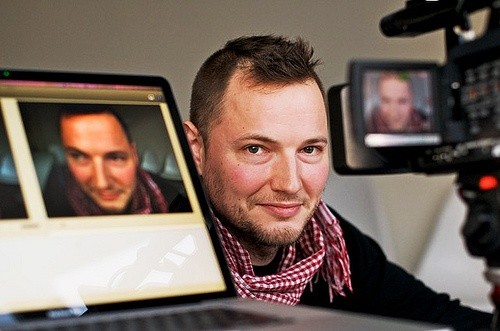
[0,69,455,331]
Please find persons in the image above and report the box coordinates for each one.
[184,34,492,331]
[364,77,429,133]
[42,103,193,218]
